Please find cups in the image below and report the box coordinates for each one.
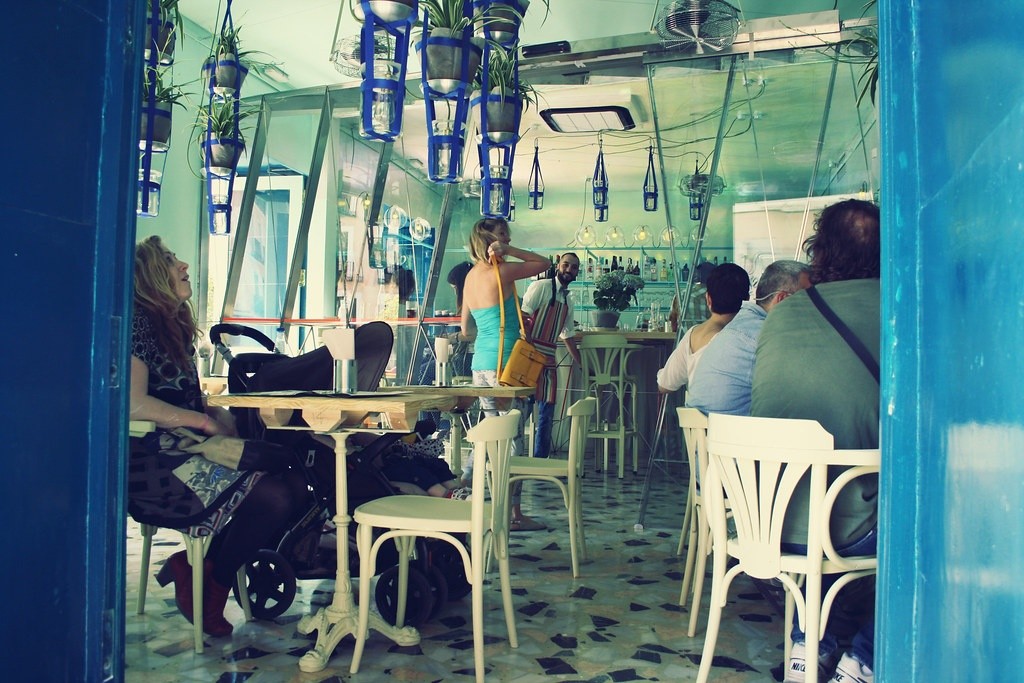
[332,358,358,395]
[435,362,452,387]
[665,321,672,333]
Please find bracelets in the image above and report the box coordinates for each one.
[198,413,208,431]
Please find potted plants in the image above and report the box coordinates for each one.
[201,24,288,96]
[186,98,261,176]
[142,0,183,67]
[348,0,554,138]
[135,67,197,154]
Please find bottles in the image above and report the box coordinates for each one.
[546,255,728,281]
[273,327,289,355]
[648,321,652,332]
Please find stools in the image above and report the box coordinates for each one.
[571,337,645,481]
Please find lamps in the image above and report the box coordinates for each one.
[632,223,655,249]
[575,225,600,250]
[684,225,711,251]
[605,225,627,250]
[353,191,377,223]
[660,224,681,248]
[384,205,409,228]
[407,218,430,244]
[336,195,347,215]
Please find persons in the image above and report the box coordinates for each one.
[128,235,308,639]
[370,434,472,499]
[747,199,880,683]
[460,217,552,531]
[419,261,474,440]
[656,260,814,416]
[512,253,596,459]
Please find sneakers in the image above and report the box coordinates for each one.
[827,650,873,683]
[783,641,844,683]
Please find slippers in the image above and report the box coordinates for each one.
[508,514,547,531]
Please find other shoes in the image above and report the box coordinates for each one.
[443,485,473,500]
[484,488,491,501]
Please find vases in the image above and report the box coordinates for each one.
[591,308,620,329]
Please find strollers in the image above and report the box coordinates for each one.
[207,322,472,630]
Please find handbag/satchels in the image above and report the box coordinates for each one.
[500,337,547,400]
[156,424,288,486]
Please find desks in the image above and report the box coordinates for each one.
[205,388,463,672]
[377,380,540,481]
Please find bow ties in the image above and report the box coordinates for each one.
[559,286,570,297]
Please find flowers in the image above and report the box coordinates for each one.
[591,268,646,313]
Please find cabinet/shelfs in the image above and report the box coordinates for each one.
[221,315,476,395]
[524,280,686,313]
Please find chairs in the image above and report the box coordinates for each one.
[129,391,595,683]
[674,396,879,683]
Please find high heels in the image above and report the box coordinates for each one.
[155,547,233,638]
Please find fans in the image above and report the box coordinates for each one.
[649,0,744,56]
